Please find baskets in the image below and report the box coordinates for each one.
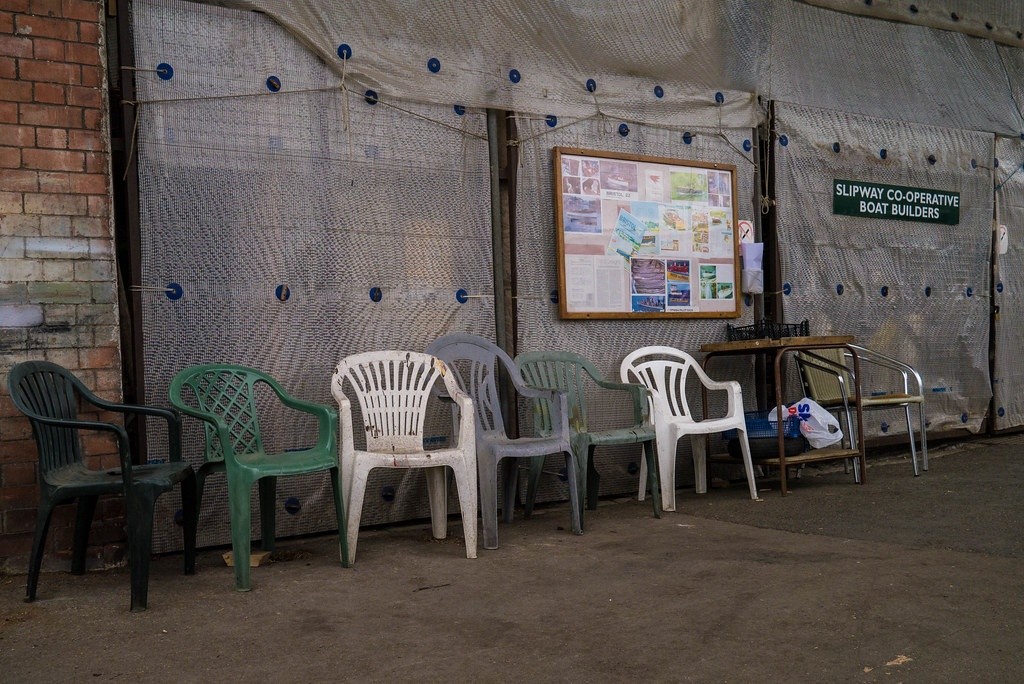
[721,409,800,439]
[727,318,811,341]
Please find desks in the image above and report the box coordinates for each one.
[700,333,866,498]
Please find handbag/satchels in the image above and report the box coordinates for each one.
[769,397,845,449]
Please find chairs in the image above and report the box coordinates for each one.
[793,342,930,484]
[619,345,758,512]
[422,332,584,550]
[8,361,200,614]
[331,349,479,565]
[168,364,353,592]
[513,351,663,531]
